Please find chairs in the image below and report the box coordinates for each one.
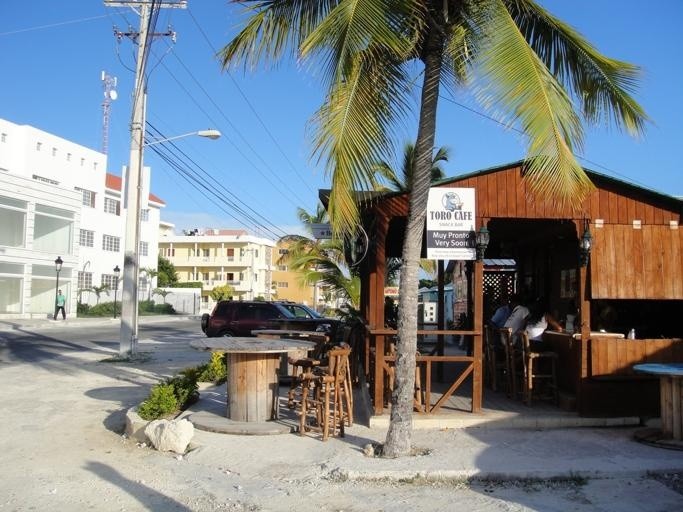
[385,325,424,414]
[187,327,366,443]
[484,322,636,407]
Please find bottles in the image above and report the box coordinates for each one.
[627,328,635,340]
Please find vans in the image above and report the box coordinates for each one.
[339,304,348,313]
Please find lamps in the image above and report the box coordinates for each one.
[576,222,595,268]
[472,219,491,262]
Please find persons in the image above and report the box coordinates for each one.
[490,290,563,397]
[54,290,66,321]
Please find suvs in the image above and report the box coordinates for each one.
[201,300,346,339]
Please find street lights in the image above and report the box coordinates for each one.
[113,265,120,319]
[132,93,220,360]
[54,255,64,319]
